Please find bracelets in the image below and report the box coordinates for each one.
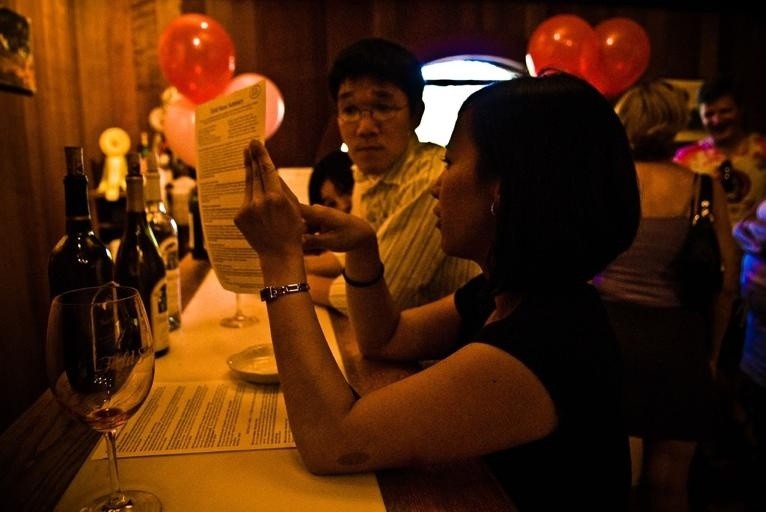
[342,263,385,288]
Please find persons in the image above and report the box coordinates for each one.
[299,38,484,317]
[592,80,740,489]
[673,77,766,228]
[234,73,640,512]
[308,147,352,215]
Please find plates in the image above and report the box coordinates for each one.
[226,346,283,383]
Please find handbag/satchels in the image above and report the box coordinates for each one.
[686,172,724,299]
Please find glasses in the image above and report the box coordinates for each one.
[338,102,407,121]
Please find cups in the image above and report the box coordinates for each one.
[219,293,259,330]
[45,285,163,512]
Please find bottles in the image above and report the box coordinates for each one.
[144,152,181,333]
[188,185,208,260]
[117,155,169,358]
[49,145,117,396]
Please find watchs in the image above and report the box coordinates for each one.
[261,282,310,302]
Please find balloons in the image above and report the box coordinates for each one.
[526,14,596,86]
[229,71,285,142]
[163,12,235,106]
[595,18,651,97]
[163,92,198,169]
